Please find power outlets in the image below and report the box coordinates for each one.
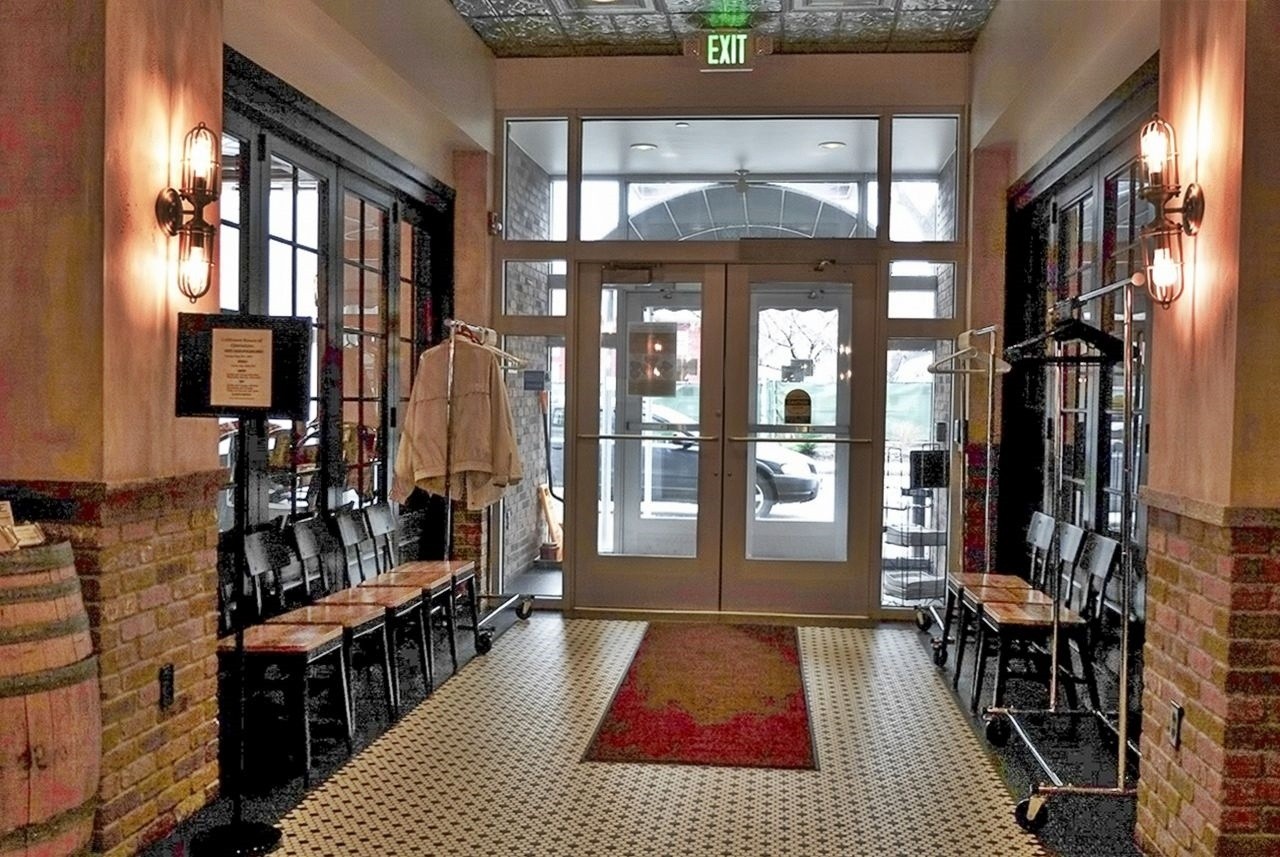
[1169,699,1185,749]
[160,664,175,706]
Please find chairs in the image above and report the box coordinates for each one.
[216,500,477,793]
[931,512,1123,729]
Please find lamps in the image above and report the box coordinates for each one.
[173,213,218,300]
[1132,116,1180,205]
[684,30,774,72]
[1139,210,1186,310]
[173,119,222,211]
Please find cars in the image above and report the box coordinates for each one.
[547,400,821,519]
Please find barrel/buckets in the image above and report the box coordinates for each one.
[0,532,104,857]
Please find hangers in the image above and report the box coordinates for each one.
[919,297,1139,372]
[446,321,529,372]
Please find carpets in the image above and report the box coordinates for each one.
[577,622,820,772]
